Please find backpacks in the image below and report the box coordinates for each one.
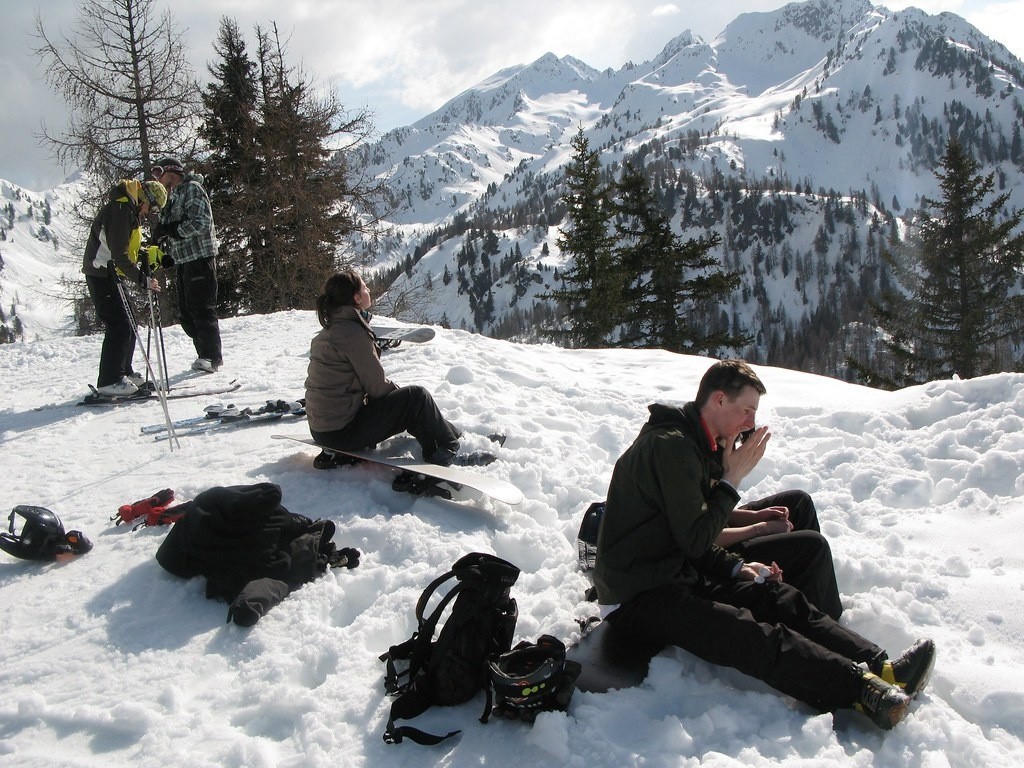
[378,552,521,746]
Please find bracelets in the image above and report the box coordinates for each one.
[752,524,759,537]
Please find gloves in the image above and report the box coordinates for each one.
[109,488,175,525]
[132,500,193,531]
[488,635,581,723]
[322,547,360,570]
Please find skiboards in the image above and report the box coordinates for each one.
[140,398,308,441]
[76,377,242,406]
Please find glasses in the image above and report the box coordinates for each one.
[150,203,162,214]
[150,166,164,179]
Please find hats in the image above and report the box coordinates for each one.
[157,158,185,176]
[139,181,167,208]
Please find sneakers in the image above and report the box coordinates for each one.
[98,376,138,396]
[127,372,144,386]
[484,432,506,448]
[452,450,494,467]
[850,671,909,731]
[868,638,936,701]
[193,358,214,373]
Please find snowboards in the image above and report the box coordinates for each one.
[564,620,657,694]
[270,432,524,507]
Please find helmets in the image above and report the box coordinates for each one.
[0,505,64,560]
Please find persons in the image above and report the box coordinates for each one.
[305,270,507,467]
[596,356,934,730]
[81,180,175,396]
[151,157,223,374]
